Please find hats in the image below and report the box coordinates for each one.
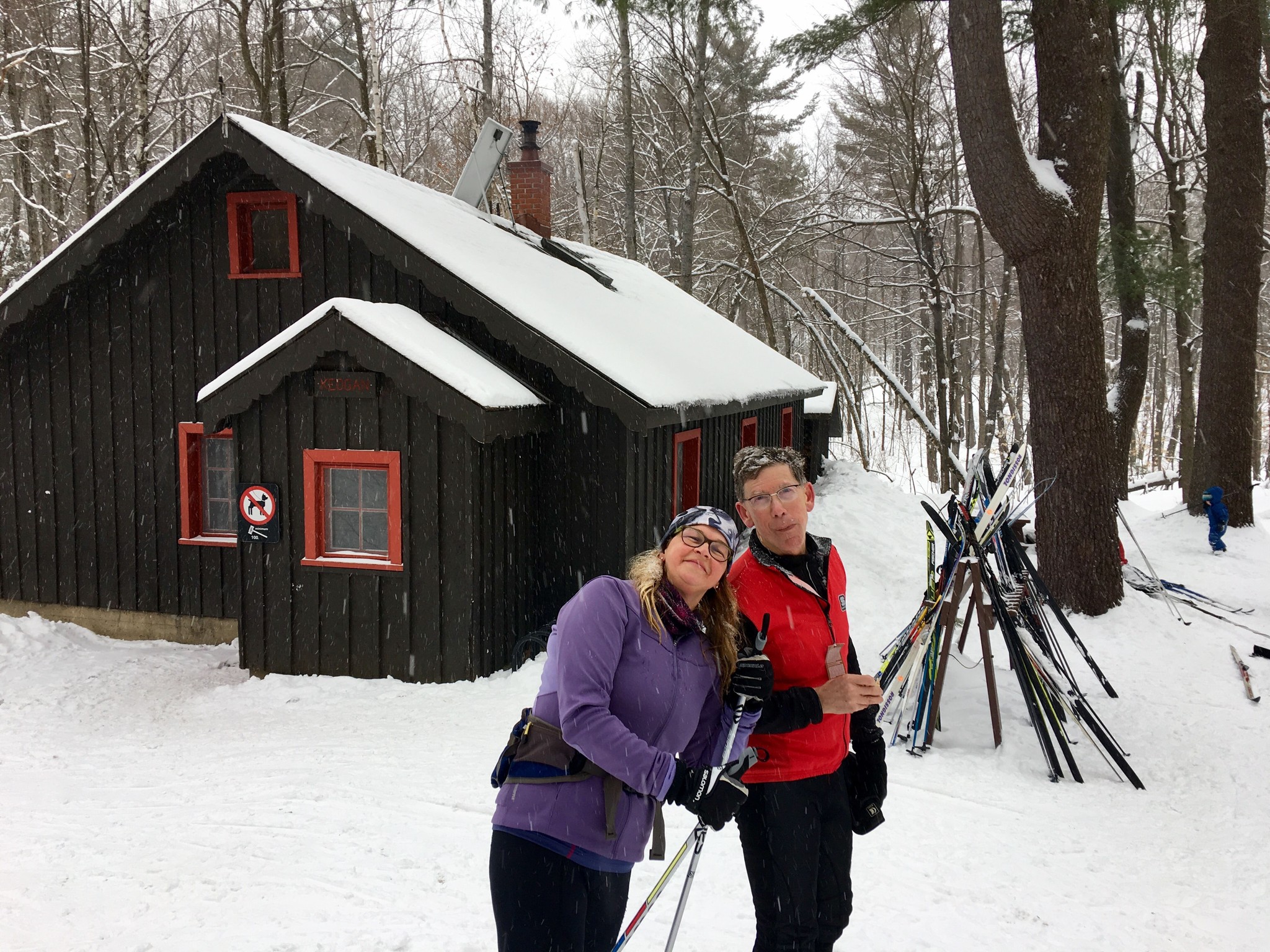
[658,506,740,574]
[842,751,885,835]
[1202,490,1212,500]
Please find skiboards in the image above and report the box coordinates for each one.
[870,445,1262,789]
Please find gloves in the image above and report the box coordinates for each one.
[726,647,774,714]
[1203,501,1210,510]
[1217,520,1228,532]
[666,758,749,832]
[849,727,887,809]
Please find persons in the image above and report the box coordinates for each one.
[488,506,775,952]
[722,445,887,952]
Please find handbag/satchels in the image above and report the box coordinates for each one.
[491,708,666,861]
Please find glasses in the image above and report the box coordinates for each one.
[741,484,804,510]
[671,524,735,562]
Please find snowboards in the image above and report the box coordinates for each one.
[1213,546,1227,556]
